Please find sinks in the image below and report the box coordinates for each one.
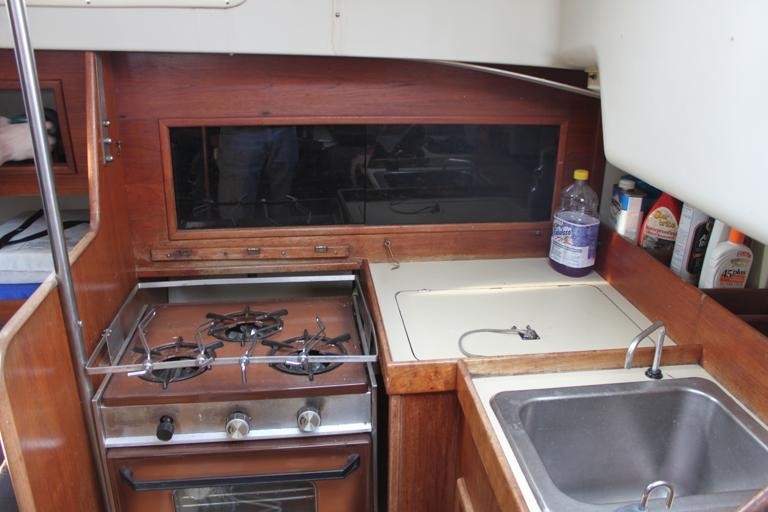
[371,169,481,190]
[489,376,768,512]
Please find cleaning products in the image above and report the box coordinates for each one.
[639,192,681,268]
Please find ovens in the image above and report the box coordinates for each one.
[99,391,380,512]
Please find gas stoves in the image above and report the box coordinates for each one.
[105,294,369,403]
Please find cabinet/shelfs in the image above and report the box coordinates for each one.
[107,440,373,510]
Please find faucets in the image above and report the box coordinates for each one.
[438,157,479,189]
[623,320,666,380]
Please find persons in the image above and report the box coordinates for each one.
[0,115,56,166]
